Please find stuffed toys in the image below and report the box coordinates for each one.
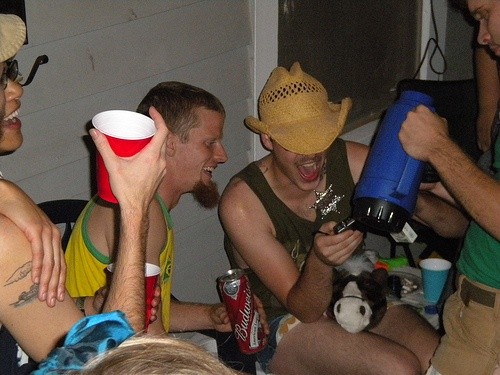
[330,258,390,333]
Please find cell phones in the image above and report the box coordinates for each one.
[334,217,359,235]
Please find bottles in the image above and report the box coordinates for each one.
[351,92,435,234]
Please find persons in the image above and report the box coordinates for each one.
[220,62,458,375]
[0,12,170,363]
[66,339,236,375]
[400,0,500,375]
[64,81,270,341]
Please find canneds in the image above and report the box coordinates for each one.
[218,269,267,354]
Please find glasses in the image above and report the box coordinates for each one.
[0,59,18,90]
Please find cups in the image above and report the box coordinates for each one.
[420,257,453,302]
[107,263,163,335]
[91,109,156,204]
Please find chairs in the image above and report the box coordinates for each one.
[389,78,481,268]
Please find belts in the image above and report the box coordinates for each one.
[455,271,496,307]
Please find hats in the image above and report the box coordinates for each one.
[0,14,26,62]
[245,62,352,155]
